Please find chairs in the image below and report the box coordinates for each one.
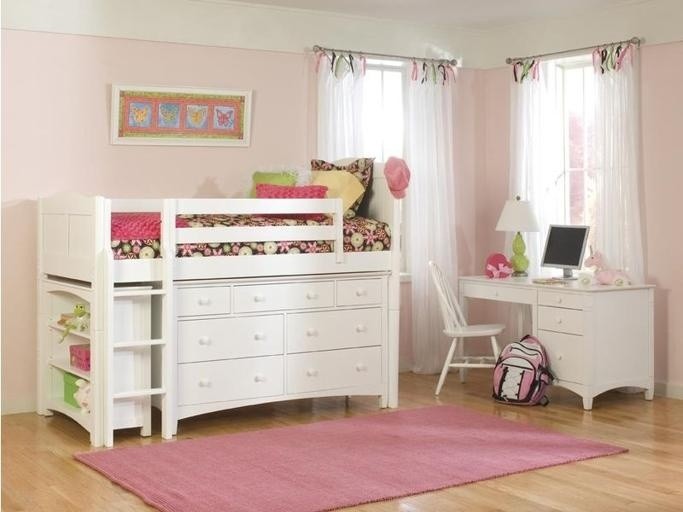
[429,260,506,396]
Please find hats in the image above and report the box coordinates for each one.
[384,157,410,199]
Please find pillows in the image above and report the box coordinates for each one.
[252,158,374,221]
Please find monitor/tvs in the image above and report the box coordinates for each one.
[540,224,590,281]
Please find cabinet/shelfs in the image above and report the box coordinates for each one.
[40,278,153,449]
[34,190,402,450]
[457,274,657,411]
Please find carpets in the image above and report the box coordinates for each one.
[73,409,630,511]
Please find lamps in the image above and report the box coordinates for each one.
[494,195,541,278]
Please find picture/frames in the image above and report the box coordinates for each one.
[111,83,253,149]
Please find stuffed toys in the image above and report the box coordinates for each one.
[57,303,91,345]
[583,245,632,285]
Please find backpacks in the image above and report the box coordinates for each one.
[492,334,554,408]
